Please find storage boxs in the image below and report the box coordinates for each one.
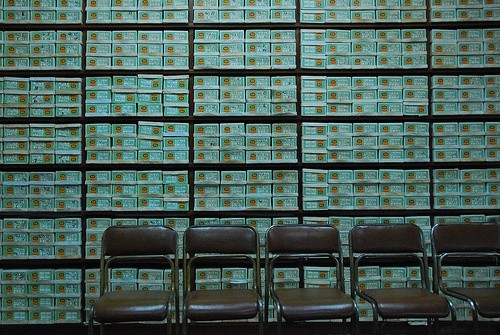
[84,0,190,323]
[428,0,500,322]
[298,0,432,322]
[0,2,82,322]
[193,0,299,319]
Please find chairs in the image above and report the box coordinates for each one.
[431,224,500,335]
[182,225,263,335]
[264,224,359,335]
[87,225,179,335]
[349,224,457,335]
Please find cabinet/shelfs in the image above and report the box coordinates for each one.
[0,0,500,325]
